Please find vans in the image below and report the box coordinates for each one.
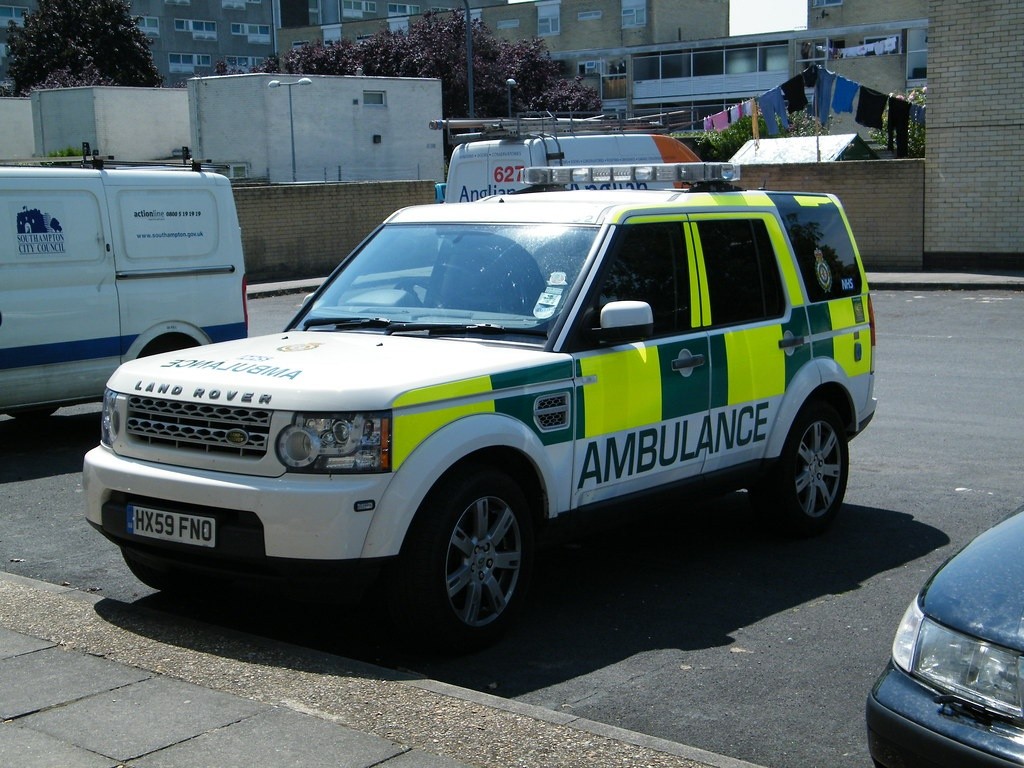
[0,167,248,418]
[430,117,704,205]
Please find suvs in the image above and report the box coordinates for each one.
[77,162,877,629]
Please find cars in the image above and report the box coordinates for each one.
[868,509,1024,768]
